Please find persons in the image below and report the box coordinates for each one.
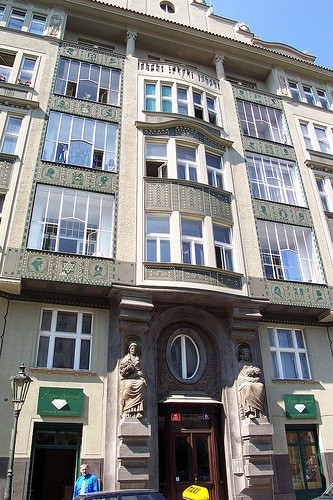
[73,462,99,498]
[120,342,148,420]
[236,346,268,419]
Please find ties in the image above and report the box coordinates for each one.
[79,479,85,496]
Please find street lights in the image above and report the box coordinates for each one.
[5,364,31,499]
